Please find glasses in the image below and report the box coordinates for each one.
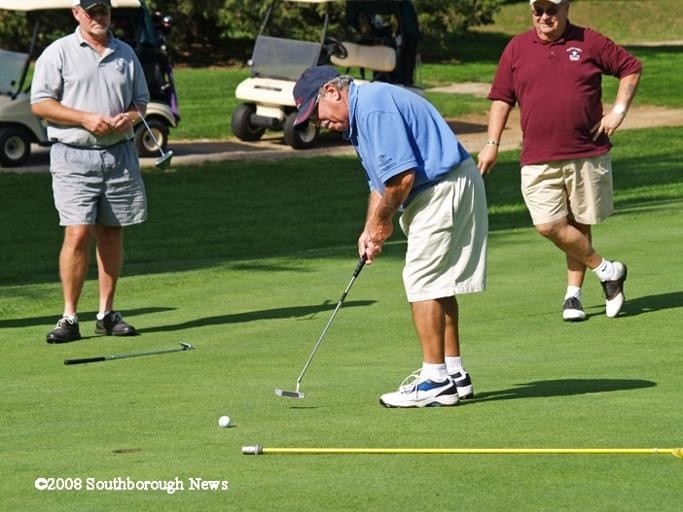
[531,6,560,17]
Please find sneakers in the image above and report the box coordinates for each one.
[562,297,585,322]
[603,262,627,318]
[381,370,473,408]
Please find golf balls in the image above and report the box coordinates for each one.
[217,416,231,425]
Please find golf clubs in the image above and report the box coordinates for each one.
[65,341,194,366]
[275,254,368,398]
[132,100,173,166]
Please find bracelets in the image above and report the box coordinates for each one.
[124,112,138,125]
[487,141,499,146]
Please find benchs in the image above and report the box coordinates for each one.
[328,40,398,85]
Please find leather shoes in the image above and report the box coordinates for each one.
[94,312,134,336]
[46,319,81,344]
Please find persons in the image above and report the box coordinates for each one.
[474,0,643,323]
[291,65,487,408]
[612,105,625,115]
[28,1,150,346]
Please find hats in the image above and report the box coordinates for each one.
[293,67,340,126]
[74,1,112,10]
[530,0,566,5]
